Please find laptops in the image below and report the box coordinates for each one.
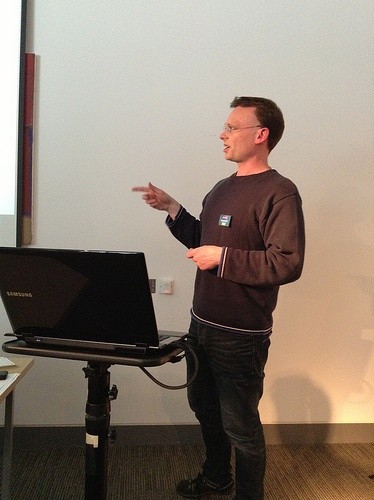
[0,246,190,357]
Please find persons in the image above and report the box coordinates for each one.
[130,94,307,498]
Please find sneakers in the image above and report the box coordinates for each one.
[177,471,234,497]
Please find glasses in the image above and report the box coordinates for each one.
[223,122,262,132]
